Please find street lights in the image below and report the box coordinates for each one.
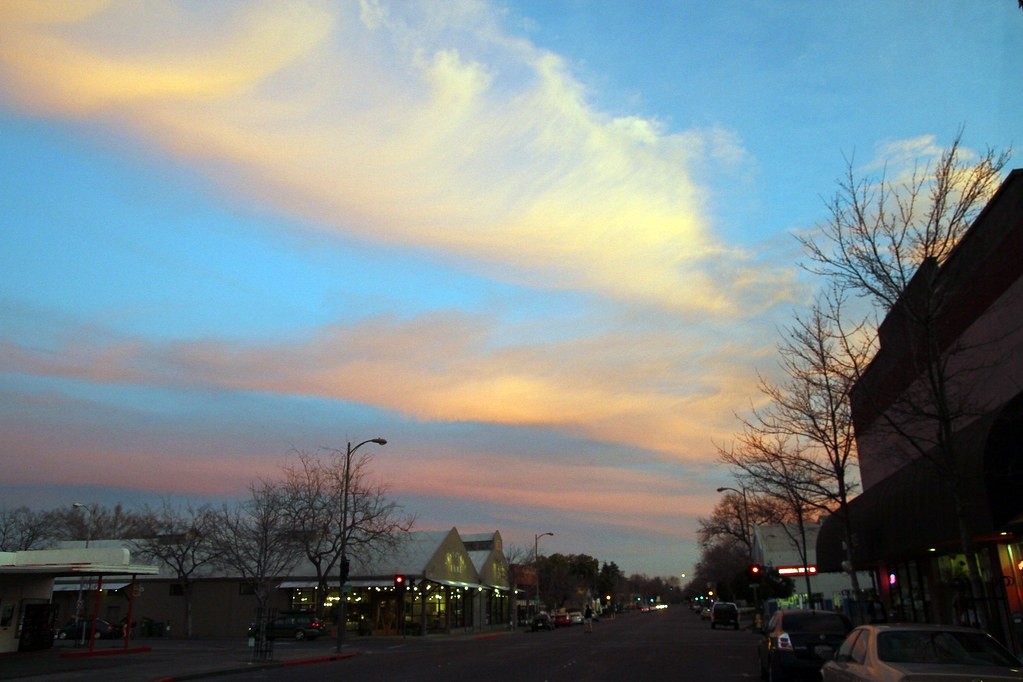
[72,502,93,549]
[535,532,553,614]
[335,437,386,652]
[717,485,758,608]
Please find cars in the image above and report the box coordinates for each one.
[532,612,585,631]
[341,609,364,632]
[688,600,739,631]
[752,608,854,682]
[815,623,1023,682]
[592,611,600,622]
[58,616,114,639]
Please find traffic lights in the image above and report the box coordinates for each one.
[707,591,714,596]
[605,594,613,600]
[749,564,762,576]
[699,595,704,600]
[694,596,699,601]
[393,575,406,586]
[636,597,641,601]
[650,597,654,605]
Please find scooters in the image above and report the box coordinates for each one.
[119,622,137,640]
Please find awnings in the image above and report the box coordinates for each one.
[53,583,131,590]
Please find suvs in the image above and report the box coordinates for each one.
[248,608,328,641]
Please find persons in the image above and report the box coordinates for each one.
[868,595,888,623]
[584,605,592,633]
[119,613,138,640]
